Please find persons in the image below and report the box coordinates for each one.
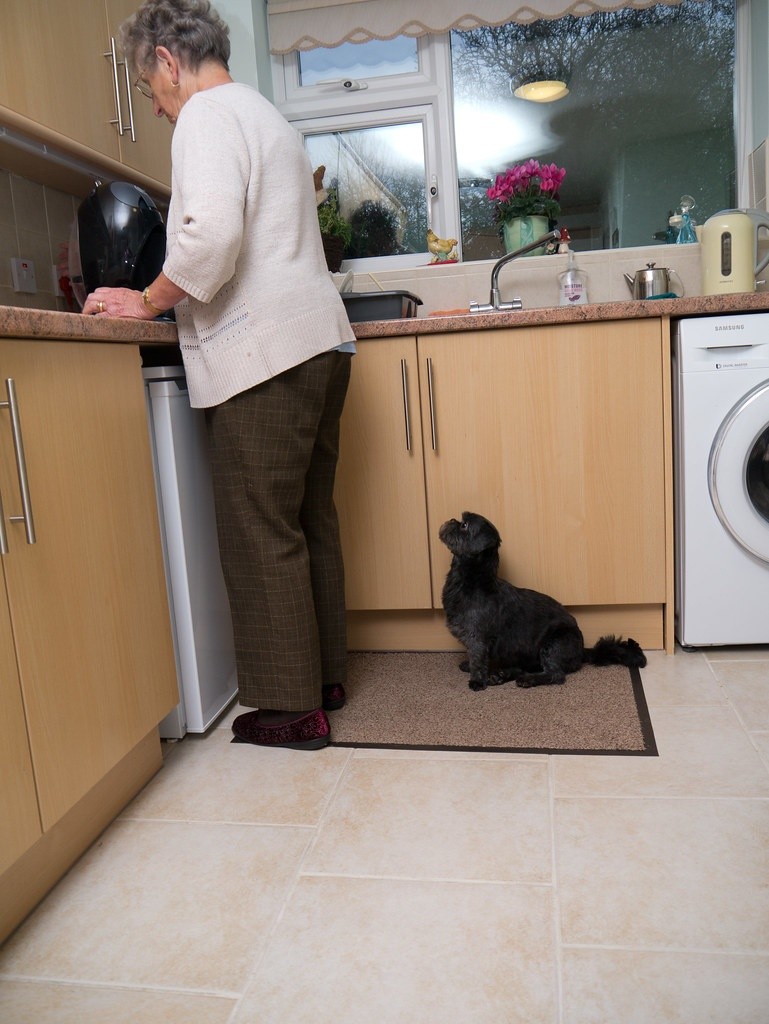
[79,2,360,752]
[668,194,699,243]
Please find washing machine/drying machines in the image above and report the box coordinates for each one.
[670,310,768,651]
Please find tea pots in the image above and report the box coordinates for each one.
[623,263,687,302]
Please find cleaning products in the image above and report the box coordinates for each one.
[555,249,591,307]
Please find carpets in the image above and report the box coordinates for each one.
[229,650,659,757]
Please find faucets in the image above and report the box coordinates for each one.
[490,228,563,311]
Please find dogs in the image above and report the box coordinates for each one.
[439,513,649,690]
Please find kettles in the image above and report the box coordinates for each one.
[692,207,769,297]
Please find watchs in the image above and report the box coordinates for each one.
[141,286,168,315]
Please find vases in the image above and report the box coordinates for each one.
[504,214,550,255]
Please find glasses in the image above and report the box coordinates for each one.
[134,55,159,100]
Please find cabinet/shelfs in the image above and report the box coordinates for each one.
[333,303,671,652]
[0,0,177,225]
[1,309,184,947]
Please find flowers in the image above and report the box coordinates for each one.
[486,160,577,217]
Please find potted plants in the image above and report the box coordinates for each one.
[313,188,352,272]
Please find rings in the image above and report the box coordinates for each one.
[97,300,104,313]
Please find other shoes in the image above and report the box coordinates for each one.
[315,684,346,712]
[232,705,333,749]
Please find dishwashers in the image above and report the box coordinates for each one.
[146,376,238,742]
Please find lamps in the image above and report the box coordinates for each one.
[510,67,569,102]
